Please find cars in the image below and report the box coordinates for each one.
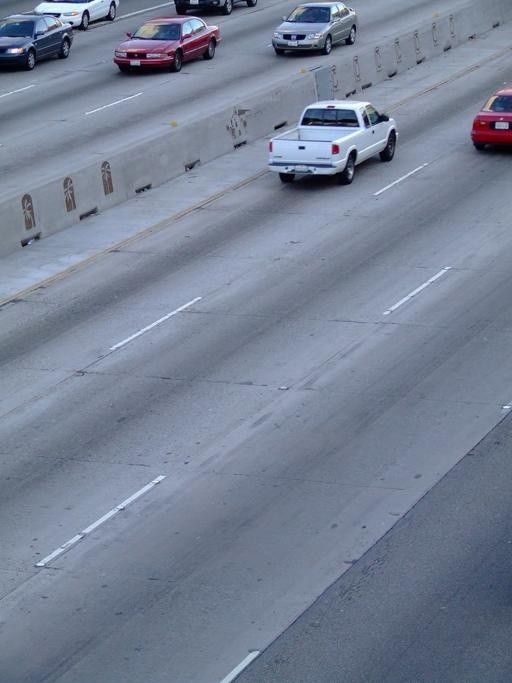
[175,0,256,13]
[0,13,73,72]
[471,86,512,152]
[272,1,357,56]
[115,14,220,73]
[34,0,120,29]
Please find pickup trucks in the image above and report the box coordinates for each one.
[269,99,398,186]
